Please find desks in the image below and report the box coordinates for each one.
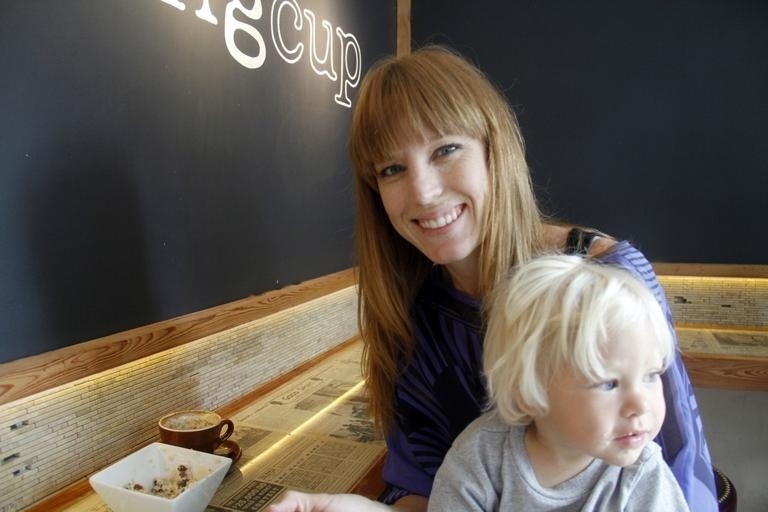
[17,322,391,512]
[671,323,768,392]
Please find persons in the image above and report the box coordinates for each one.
[263,43,722,512]
[423,250,693,512]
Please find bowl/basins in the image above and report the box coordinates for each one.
[87,441,232,512]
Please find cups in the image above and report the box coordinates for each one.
[158,411,233,453]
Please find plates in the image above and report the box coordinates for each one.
[214,443,242,463]
